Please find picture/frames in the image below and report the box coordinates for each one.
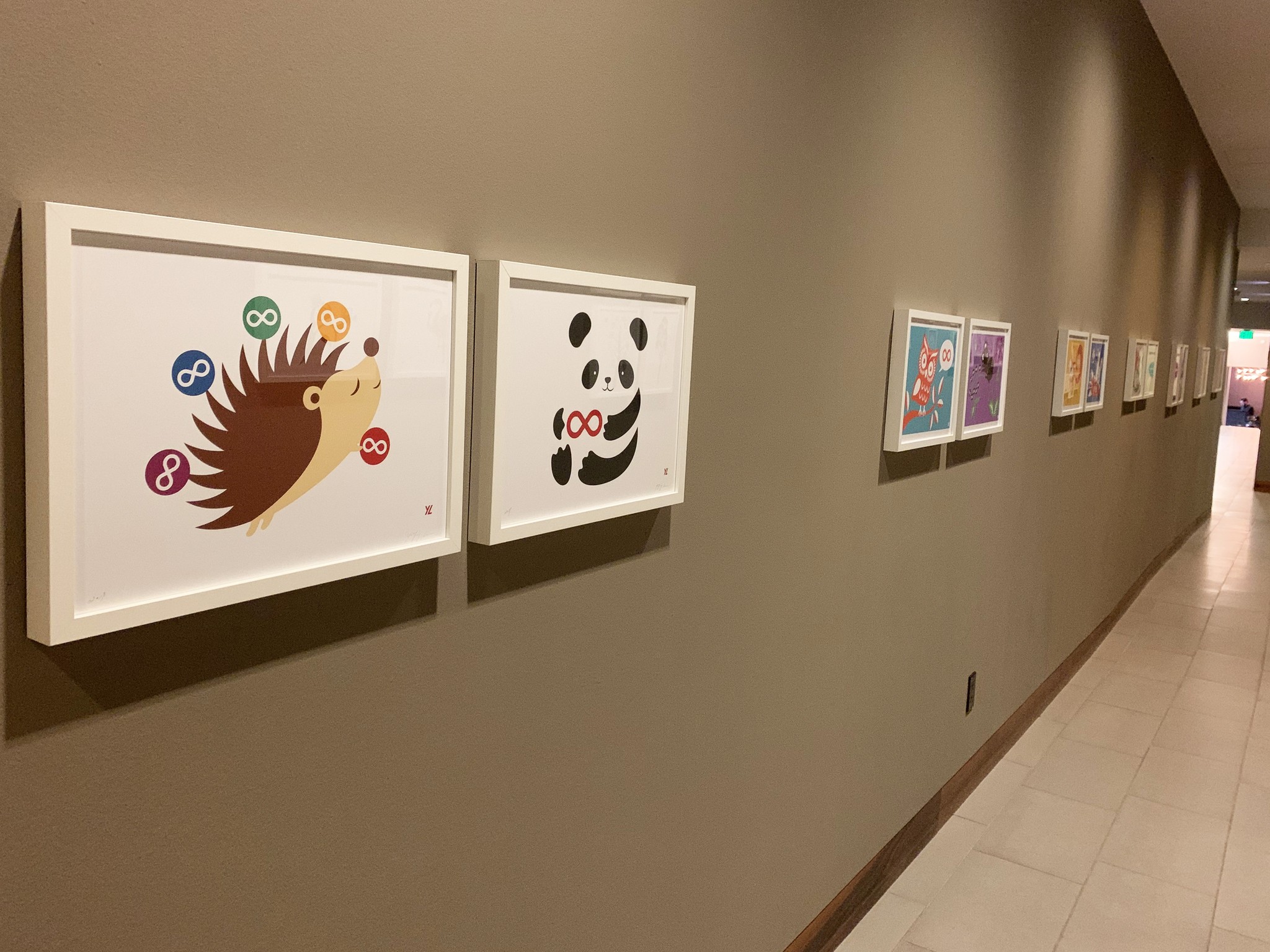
[1123,338,1148,402]
[1051,329,1090,418]
[470,255,696,549]
[1166,343,1189,408]
[1212,348,1226,392]
[882,309,966,453]
[1083,333,1109,413]
[1193,346,1211,401]
[955,316,1012,440]
[21,199,470,648]
[1142,339,1159,400]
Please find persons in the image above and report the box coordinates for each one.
[1240,398,1254,422]
